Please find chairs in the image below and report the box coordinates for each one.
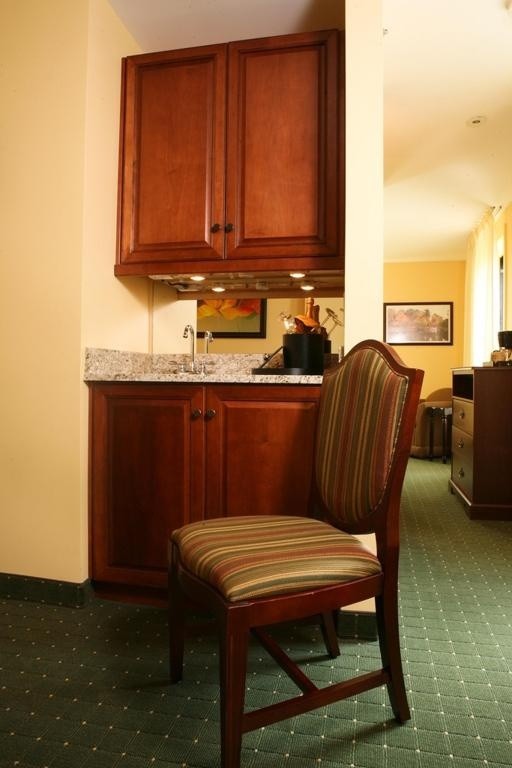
[163,331,428,767]
[406,387,454,461]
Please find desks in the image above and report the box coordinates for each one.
[421,405,455,463]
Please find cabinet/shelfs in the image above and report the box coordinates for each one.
[86,376,324,614]
[444,365,511,525]
[111,25,343,298]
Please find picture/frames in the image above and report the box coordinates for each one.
[196,298,267,340]
[381,300,454,348]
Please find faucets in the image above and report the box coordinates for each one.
[202,328,214,354]
[182,324,198,371]
